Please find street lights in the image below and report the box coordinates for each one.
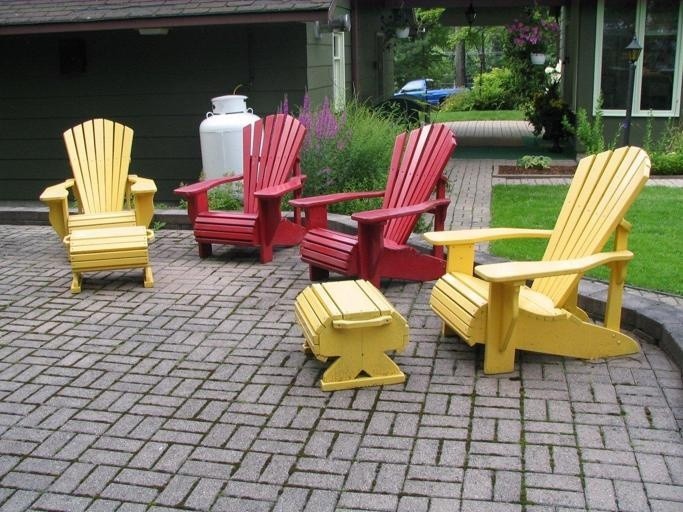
[622,34,644,147]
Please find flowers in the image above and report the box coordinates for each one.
[504,1,561,53]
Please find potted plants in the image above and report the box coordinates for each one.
[382,12,426,39]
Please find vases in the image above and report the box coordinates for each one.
[530,51,546,65]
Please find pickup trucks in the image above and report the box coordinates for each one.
[392,77,470,108]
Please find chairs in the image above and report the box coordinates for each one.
[423,145,652,376]
[173,113,308,264]
[288,123,457,291]
[39,118,158,262]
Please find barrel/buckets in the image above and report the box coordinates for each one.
[199,95,264,200]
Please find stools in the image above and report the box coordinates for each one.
[294,279,410,391]
[63,225,154,293]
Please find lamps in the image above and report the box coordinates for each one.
[465,2,478,30]
